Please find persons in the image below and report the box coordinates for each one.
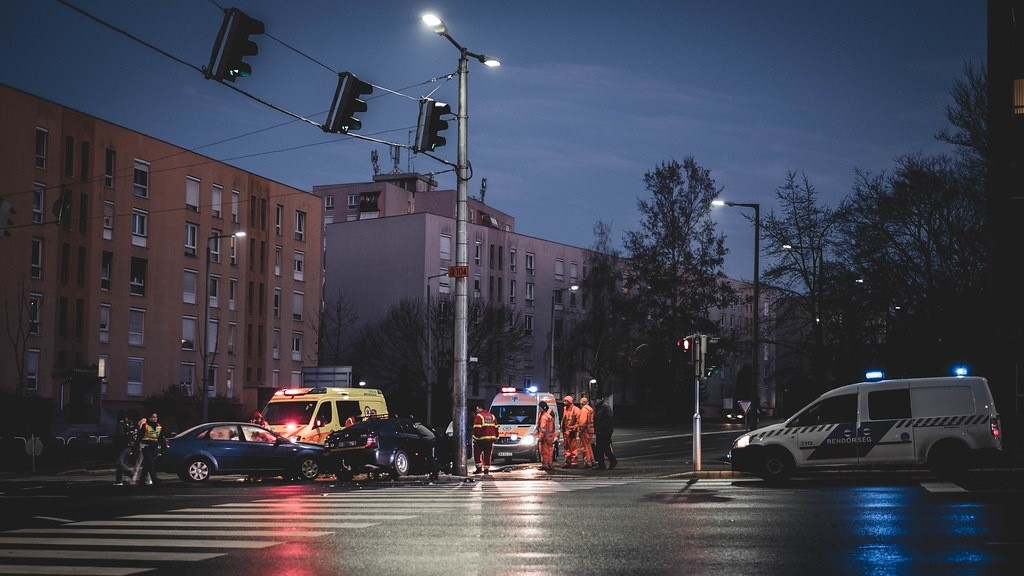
[113,411,167,487]
[366,409,381,421]
[537,402,555,471]
[472,403,499,474]
[573,397,597,469]
[346,410,362,428]
[559,396,581,469]
[254,413,265,427]
[413,421,439,481]
[591,395,617,470]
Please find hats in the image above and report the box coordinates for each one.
[591,395,602,400]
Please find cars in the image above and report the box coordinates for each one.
[157,422,335,484]
[323,413,455,483]
[724,404,761,424]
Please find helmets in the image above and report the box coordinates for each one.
[563,396,573,404]
[538,401,549,412]
[580,398,589,405]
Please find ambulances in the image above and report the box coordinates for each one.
[487,385,561,464]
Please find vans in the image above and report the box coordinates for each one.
[253,386,390,448]
[726,366,1004,485]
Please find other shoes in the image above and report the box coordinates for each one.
[473,468,482,474]
[608,460,618,470]
[484,469,488,474]
[144,480,153,485]
[125,477,136,485]
[113,481,123,485]
[592,464,605,470]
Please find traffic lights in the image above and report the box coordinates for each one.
[700,334,721,378]
[323,71,374,135]
[677,336,696,363]
[414,99,452,154]
[213,9,266,84]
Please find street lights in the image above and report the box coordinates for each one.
[419,10,504,478]
[201,231,247,423]
[548,285,580,395]
[781,243,824,395]
[711,200,761,430]
